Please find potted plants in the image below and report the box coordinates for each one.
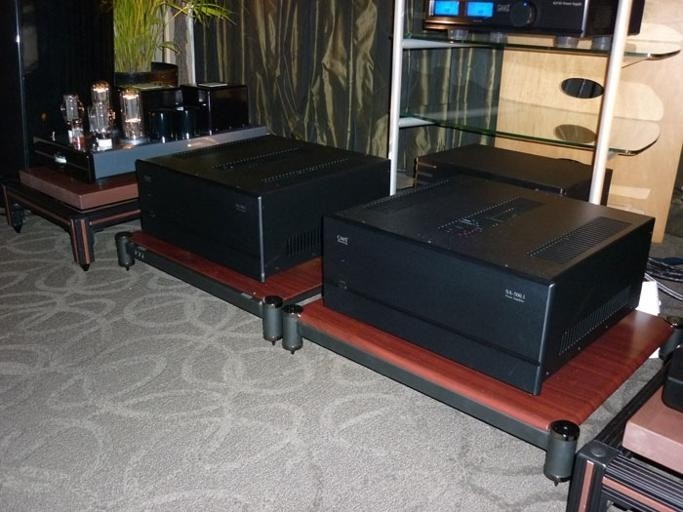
[112,1,236,93]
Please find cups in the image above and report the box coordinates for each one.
[65,95,79,125]
[71,118,85,152]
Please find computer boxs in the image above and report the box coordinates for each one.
[413,143,614,208]
[135,135,390,285]
[321,173,656,398]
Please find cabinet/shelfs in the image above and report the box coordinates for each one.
[382,0,683,208]
